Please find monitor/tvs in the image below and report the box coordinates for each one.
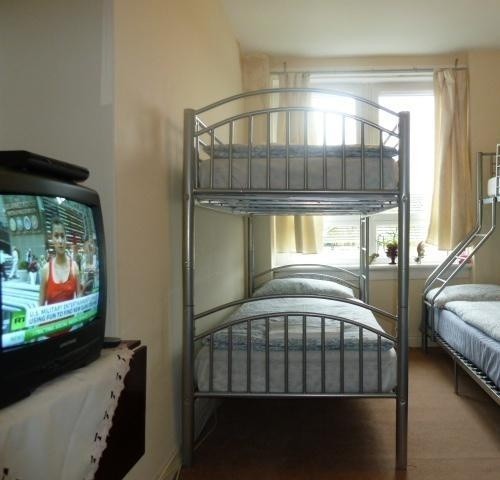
[0,149,108,413]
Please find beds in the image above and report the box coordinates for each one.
[180,88,412,218]
[181,264,410,475]
[418,146,500,408]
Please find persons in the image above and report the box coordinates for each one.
[37,219,93,337]
[0,245,33,287]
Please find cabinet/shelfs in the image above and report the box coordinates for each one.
[0,339,147,479]
